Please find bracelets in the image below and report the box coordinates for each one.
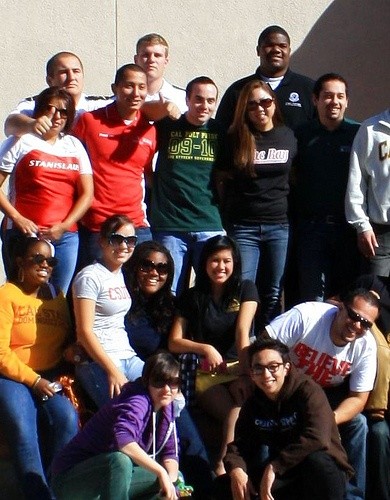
[32,378,41,392]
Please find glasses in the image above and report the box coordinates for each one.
[22,255,57,267]
[150,375,181,389]
[344,303,373,330]
[42,103,68,120]
[246,97,275,112]
[250,361,285,375]
[101,232,138,248]
[135,257,169,276]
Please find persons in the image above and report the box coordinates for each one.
[284,73,362,311]
[344,108,390,339]
[0,52,182,340]
[214,25,318,126]
[150,76,227,296]
[212,288,390,500]
[0,213,260,500]
[223,80,297,343]
[110,33,194,171]
